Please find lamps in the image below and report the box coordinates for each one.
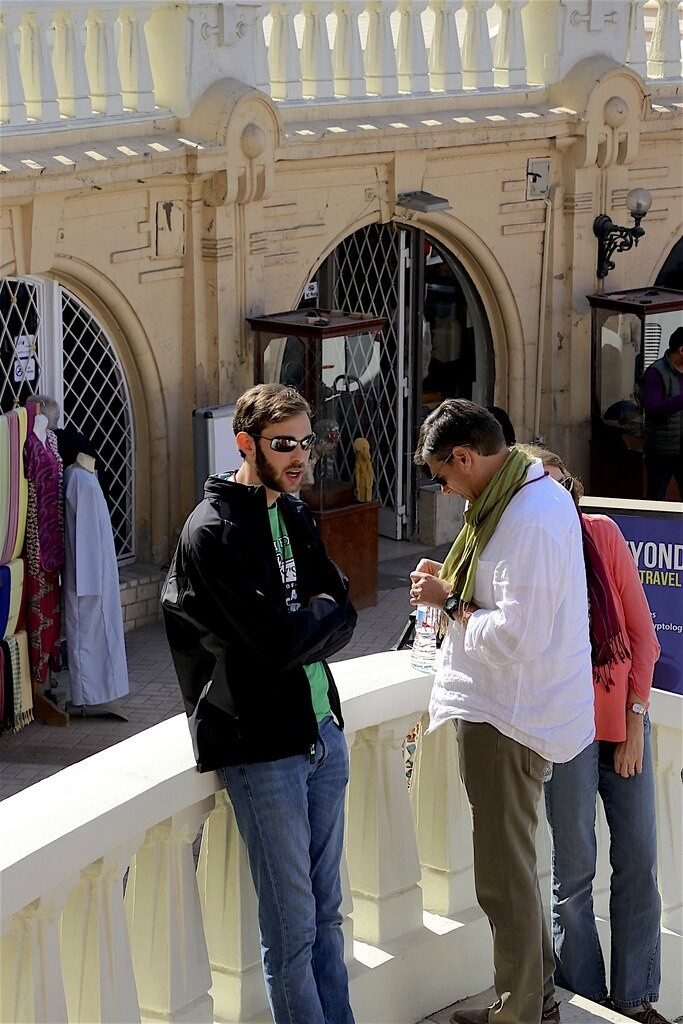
[398,192,452,213]
[594,188,651,278]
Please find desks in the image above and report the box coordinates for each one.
[308,499,382,609]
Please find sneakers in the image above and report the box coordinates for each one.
[449,998,562,1024]
[628,1000,671,1024]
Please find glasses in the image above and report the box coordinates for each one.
[429,444,470,485]
[560,477,573,492]
[251,433,315,450]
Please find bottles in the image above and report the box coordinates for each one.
[543,760,553,783]
[410,604,440,673]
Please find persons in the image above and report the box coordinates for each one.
[410,398,596,1024]
[22,414,129,707]
[640,326,683,503]
[511,445,673,1024]
[158,382,355,1024]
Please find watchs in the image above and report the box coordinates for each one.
[444,594,461,620]
[627,702,646,715]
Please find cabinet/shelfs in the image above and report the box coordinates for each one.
[584,286,683,501]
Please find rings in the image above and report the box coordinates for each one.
[410,590,415,597]
